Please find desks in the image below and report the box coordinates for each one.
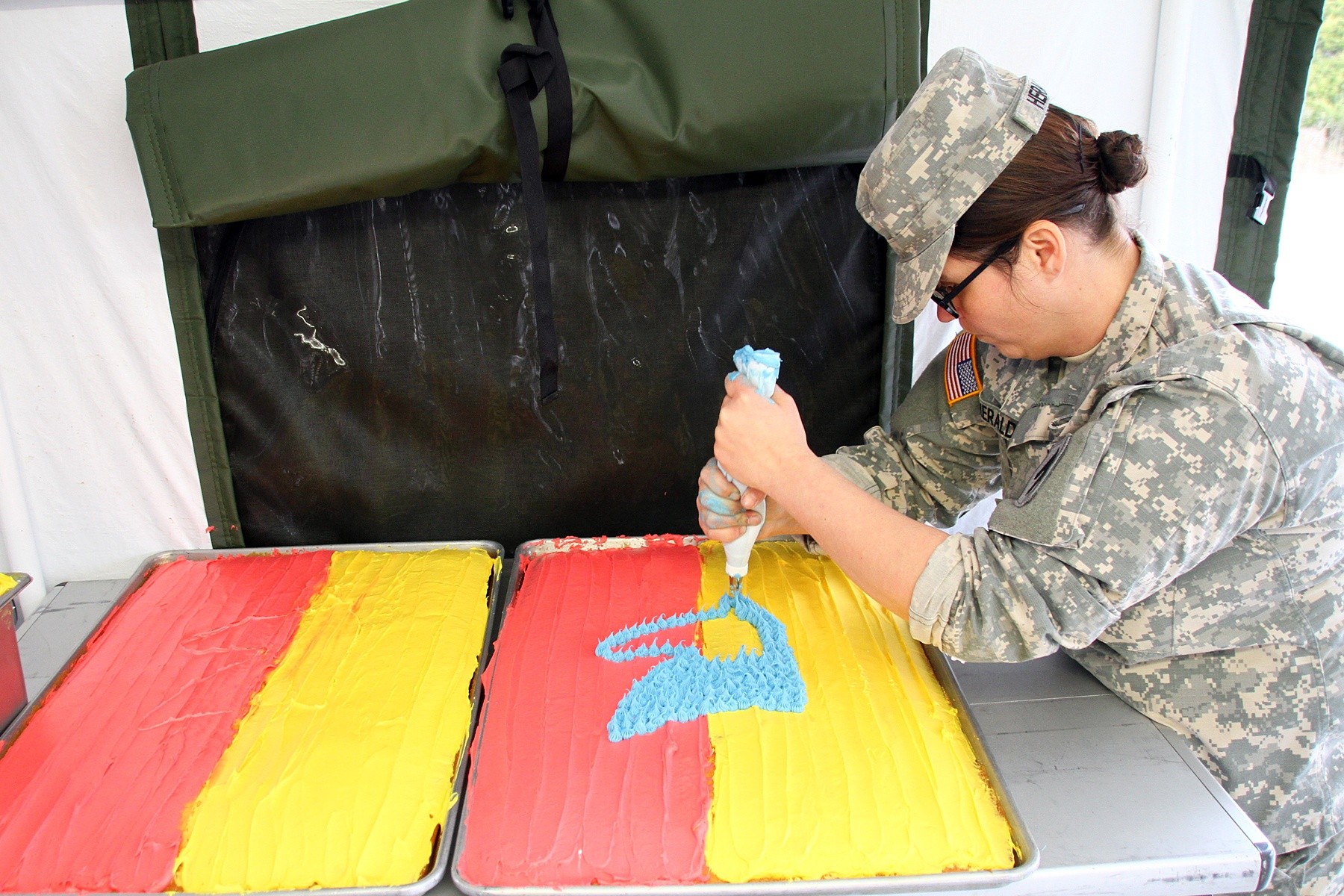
[12,577,1276,896]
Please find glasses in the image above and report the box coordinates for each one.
[930,204,1085,319]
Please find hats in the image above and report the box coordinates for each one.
[853,45,1049,324]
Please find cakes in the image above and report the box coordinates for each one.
[457,541,1023,888]
[0,549,494,896]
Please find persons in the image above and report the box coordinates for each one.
[694,47,1344,896]
